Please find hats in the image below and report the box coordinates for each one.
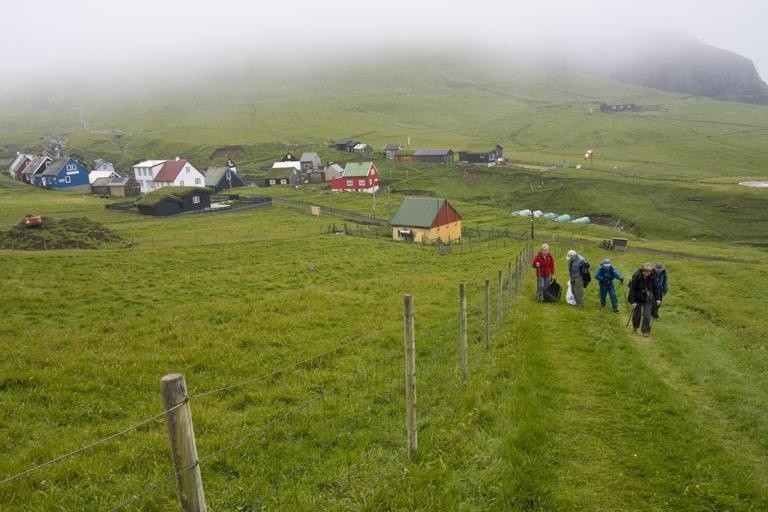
[603,259,613,267]
[566,250,577,261]
[639,262,666,272]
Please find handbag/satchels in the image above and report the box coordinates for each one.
[543,280,562,303]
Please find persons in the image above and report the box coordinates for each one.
[531,243,555,302]
[595,258,624,313]
[564,250,589,305]
[626,263,661,337]
[649,263,669,320]
